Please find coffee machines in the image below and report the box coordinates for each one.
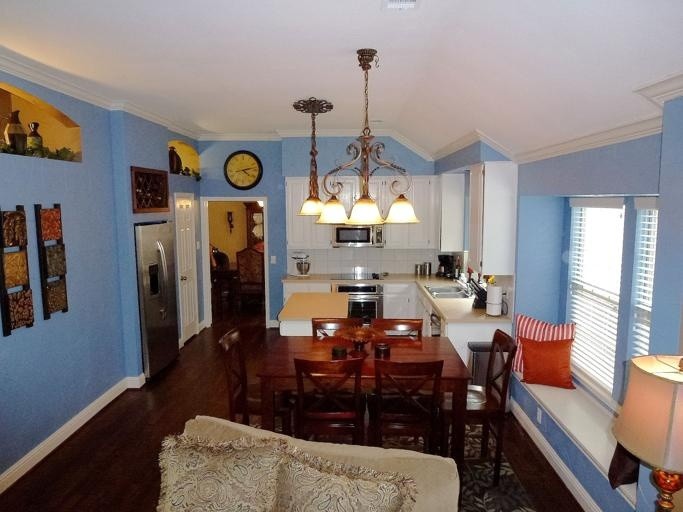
[435,255,455,279]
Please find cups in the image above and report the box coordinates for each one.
[375,342,391,361]
[332,344,347,360]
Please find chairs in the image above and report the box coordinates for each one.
[219,326,289,433]
[367,359,442,457]
[293,356,365,446]
[437,329,517,489]
[311,317,359,339]
[233,247,264,310]
[370,319,422,339]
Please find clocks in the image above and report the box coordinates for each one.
[223,149,264,190]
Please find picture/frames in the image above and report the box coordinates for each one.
[130,164,171,213]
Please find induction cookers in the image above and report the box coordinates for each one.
[330,273,384,280]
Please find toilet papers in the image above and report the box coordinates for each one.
[486,285,502,315]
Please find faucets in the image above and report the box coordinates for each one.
[461,279,469,293]
[453,280,465,289]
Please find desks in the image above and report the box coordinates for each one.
[277,290,350,337]
[257,334,471,466]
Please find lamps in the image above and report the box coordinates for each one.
[297,115,327,218]
[618,352,683,512]
[314,71,424,226]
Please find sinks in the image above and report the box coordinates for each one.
[424,286,462,293]
[430,293,470,298]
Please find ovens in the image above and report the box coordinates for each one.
[336,284,383,326]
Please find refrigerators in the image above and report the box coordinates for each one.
[134,221,180,384]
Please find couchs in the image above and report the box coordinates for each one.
[178,414,461,511]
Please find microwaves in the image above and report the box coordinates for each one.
[332,223,384,249]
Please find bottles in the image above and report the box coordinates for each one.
[415,262,432,276]
[455,255,462,279]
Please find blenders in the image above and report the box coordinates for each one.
[290,254,311,277]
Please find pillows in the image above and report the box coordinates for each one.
[512,312,577,371]
[519,336,576,390]
[274,444,418,511]
[156,433,292,511]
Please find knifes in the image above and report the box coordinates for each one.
[468,278,482,294]
